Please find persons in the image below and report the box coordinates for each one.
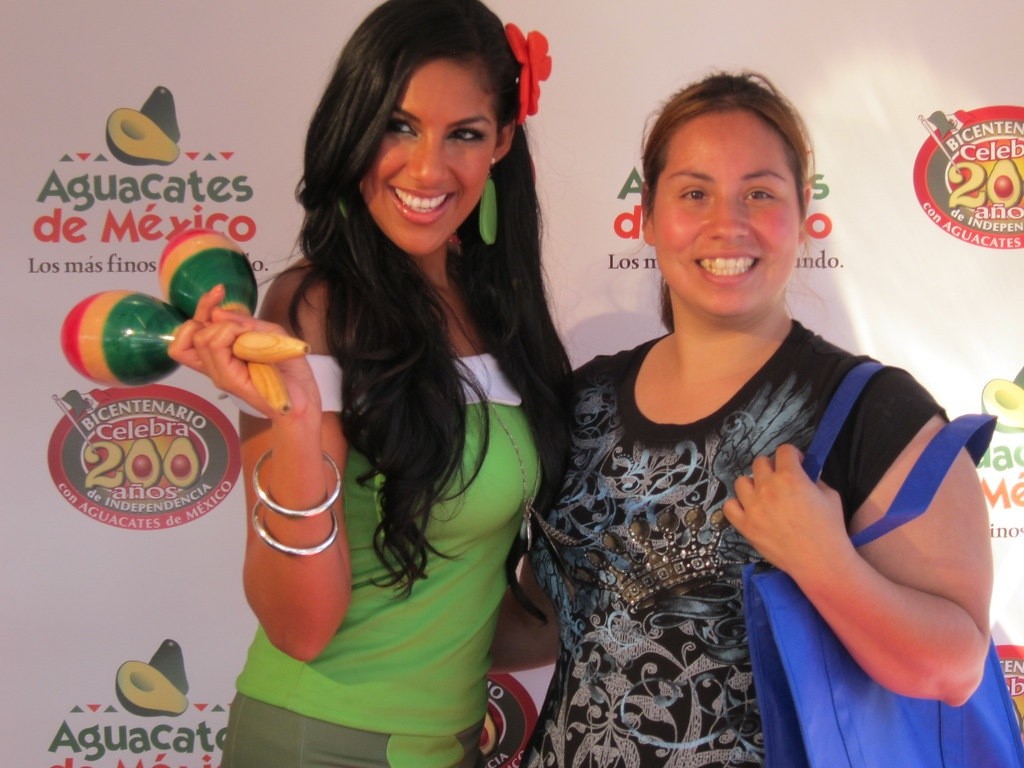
[485,72,994,768]
[168,1,579,767]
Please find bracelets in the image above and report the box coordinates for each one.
[251,498,339,557]
[253,441,343,518]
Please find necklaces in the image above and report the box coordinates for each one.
[488,395,581,555]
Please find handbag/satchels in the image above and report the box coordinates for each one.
[740,361,1024,768]
